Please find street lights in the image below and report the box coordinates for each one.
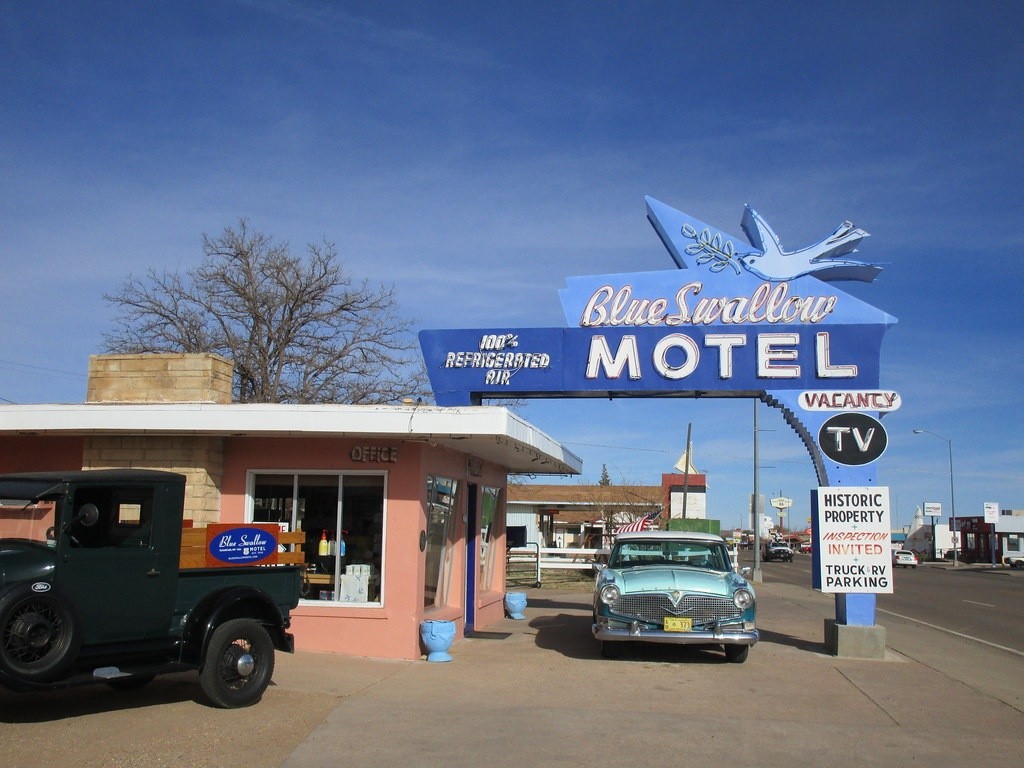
[912,430,958,568]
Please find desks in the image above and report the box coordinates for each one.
[300,572,379,602]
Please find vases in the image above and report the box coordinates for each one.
[420,621,455,662]
[504,591,528,619]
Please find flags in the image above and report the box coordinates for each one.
[615,509,662,533]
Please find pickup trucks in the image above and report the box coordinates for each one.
[0,465,305,724]
[1003,555,1024,569]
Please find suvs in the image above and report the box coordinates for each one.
[764,541,795,564]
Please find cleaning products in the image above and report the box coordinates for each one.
[340,530,348,556]
[319,530,328,556]
[328,530,336,556]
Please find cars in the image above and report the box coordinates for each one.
[591,532,759,664]
[892,549,918,570]
